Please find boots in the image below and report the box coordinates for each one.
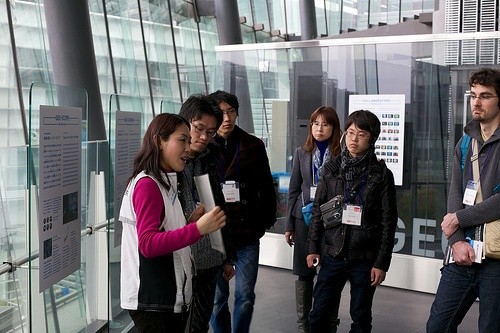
[295,281,314,333]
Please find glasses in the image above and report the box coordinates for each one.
[312,122,331,129]
[223,110,235,115]
[347,131,372,139]
[471,95,497,100]
[190,122,216,136]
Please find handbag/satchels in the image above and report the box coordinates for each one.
[320,195,345,228]
[482,220,500,258]
[302,202,314,226]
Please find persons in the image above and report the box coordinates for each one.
[207,90,277,333]
[178,92,236,333]
[285,105,344,333]
[303,109,397,333]
[118,113,227,333]
[426,67,500,333]
